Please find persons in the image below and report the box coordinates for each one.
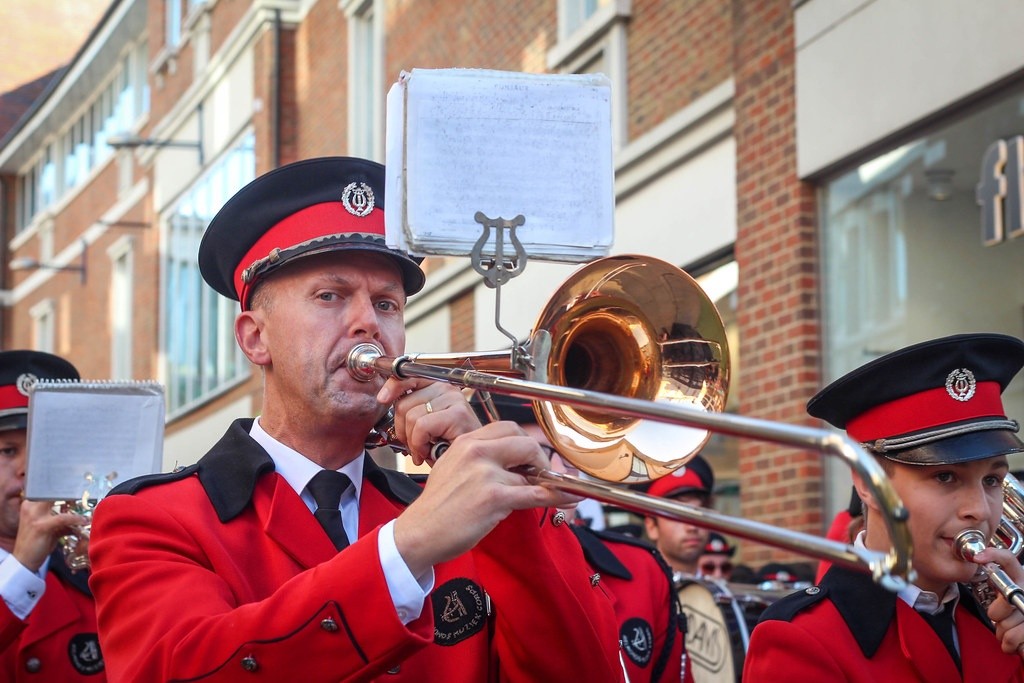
[466,386,581,522]
[627,454,714,580]
[0,348,105,683]
[85,158,625,683]
[743,333,1024,683]
[700,531,736,582]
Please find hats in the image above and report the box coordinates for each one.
[0,349,80,433]
[626,456,714,518]
[805,333,1024,466]
[468,389,538,427]
[702,532,735,557]
[198,156,426,312]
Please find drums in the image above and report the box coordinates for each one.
[673,571,815,683]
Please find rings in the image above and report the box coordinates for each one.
[426,401,433,414]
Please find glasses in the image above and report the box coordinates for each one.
[700,561,733,576]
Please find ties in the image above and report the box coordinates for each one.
[306,470,352,554]
[917,598,961,683]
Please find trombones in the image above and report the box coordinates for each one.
[350,255,912,578]
